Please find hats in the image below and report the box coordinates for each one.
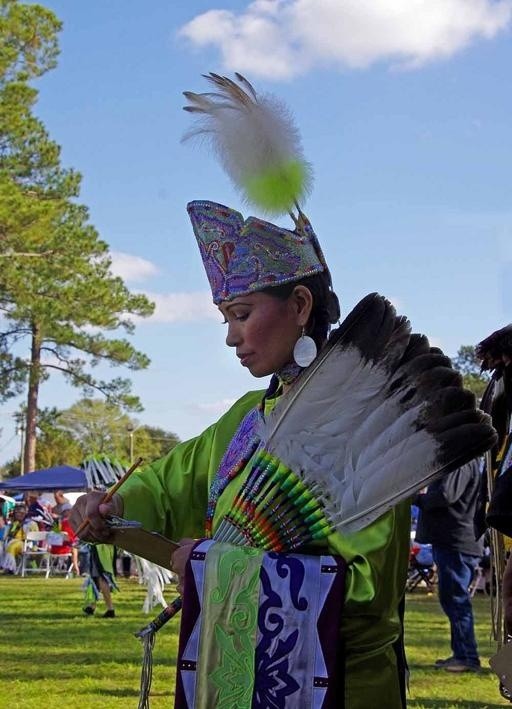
[186,200,328,305]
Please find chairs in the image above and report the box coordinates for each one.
[22,531,49,579]
[407,539,435,591]
[12,553,23,575]
[49,532,79,579]
[468,563,489,597]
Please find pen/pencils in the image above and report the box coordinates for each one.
[74,457,142,538]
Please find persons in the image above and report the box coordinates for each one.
[67,266,408,708]
[1,488,83,578]
[411,455,485,674]
[83,540,121,616]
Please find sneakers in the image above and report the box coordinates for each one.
[435,656,480,672]
[101,610,115,617]
[82,606,94,615]
